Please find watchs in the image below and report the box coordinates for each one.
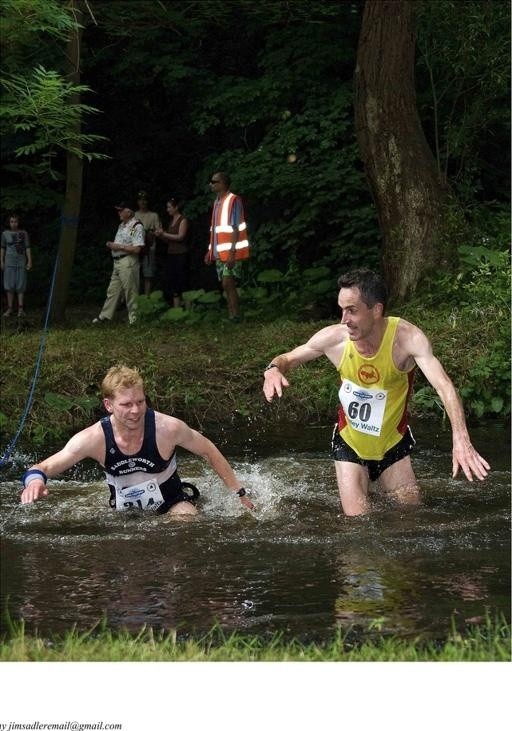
[264,363,280,372]
[236,487,246,497]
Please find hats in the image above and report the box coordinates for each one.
[114,201,138,212]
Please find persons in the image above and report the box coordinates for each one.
[134,191,163,296]
[204,171,251,324]
[262,267,491,516]
[0,213,33,319]
[20,363,257,522]
[153,200,190,310]
[92,201,147,325]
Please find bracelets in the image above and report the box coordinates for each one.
[23,469,48,488]
